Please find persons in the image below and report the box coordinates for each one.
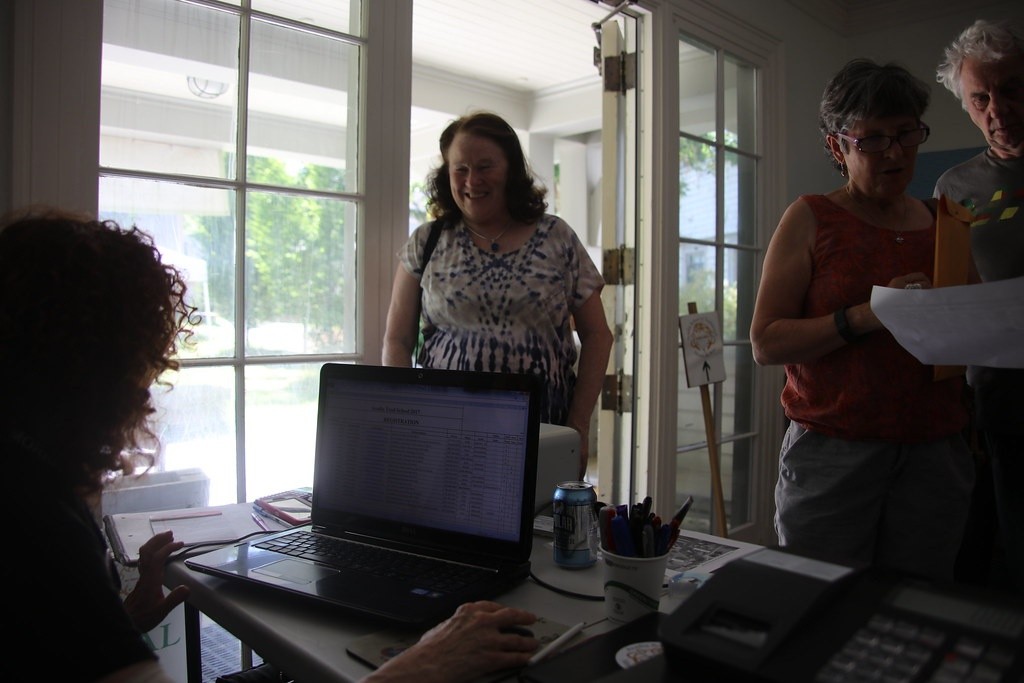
[931,21,1024,600]
[379,115,614,486]
[0,212,542,682]
[747,56,988,603]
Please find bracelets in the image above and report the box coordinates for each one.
[833,304,859,344]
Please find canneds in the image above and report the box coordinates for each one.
[552,481,601,569]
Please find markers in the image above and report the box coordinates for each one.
[255,499,298,525]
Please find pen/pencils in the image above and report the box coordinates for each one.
[594,496,694,557]
[527,622,588,665]
[251,512,271,532]
[253,506,292,527]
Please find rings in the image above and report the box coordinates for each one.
[903,282,922,292]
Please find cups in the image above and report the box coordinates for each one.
[597,545,671,623]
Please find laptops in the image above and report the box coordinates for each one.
[182,361,544,632]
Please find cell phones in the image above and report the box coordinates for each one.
[252,496,312,527]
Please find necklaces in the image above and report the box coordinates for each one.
[844,183,908,245]
[466,213,518,252]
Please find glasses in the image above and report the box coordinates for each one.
[834,118,931,154]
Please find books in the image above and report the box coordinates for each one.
[149,512,238,546]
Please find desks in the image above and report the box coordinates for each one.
[139,488,1023,681]
